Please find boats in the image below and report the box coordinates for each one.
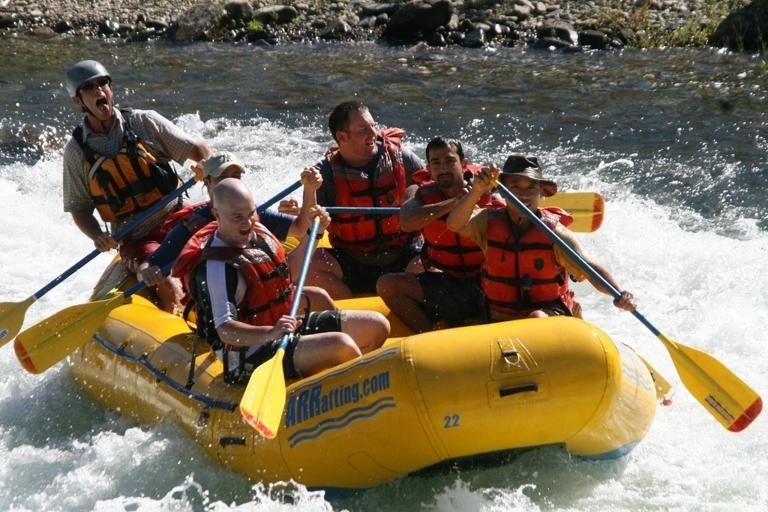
[67,199,662,491]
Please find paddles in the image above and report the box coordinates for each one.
[14,172,301,373]
[484,163,763,434]
[321,192,604,233]
[0,162,205,346]
[240,216,322,439]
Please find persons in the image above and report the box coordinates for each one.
[445,156,637,321]
[192,178,393,387]
[373,137,508,333]
[135,148,325,336]
[278,102,425,300]
[62,59,213,316]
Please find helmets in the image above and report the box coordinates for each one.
[64,57,113,100]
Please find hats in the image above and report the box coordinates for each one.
[496,153,557,199]
[202,150,246,179]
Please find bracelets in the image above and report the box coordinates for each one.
[306,230,325,241]
